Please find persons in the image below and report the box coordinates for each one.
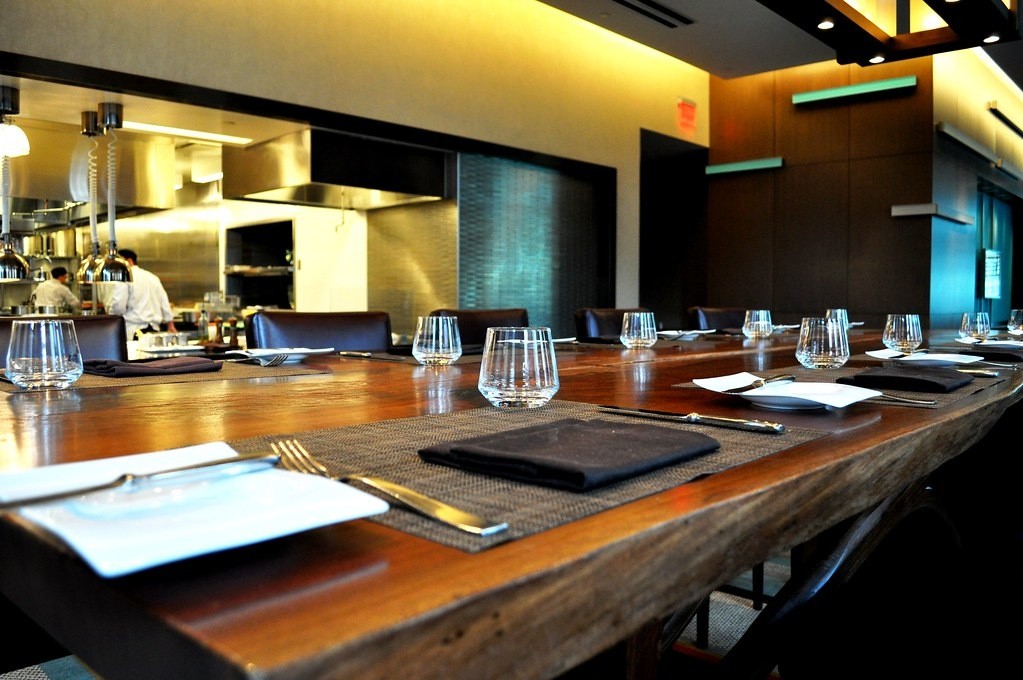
[30,267,80,313]
[109,248,178,341]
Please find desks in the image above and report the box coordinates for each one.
[0,321,1023,680]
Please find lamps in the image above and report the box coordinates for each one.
[0,86,29,282]
[75,101,132,284]
[755,0,1023,67]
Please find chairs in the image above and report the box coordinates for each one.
[0,306,1023,680]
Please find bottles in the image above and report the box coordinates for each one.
[135,311,250,351]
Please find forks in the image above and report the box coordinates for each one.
[268,437,507,538]
[226,354,288,367]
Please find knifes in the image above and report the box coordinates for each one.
[340,350,406,360]
[1,453,279,513]
[722,375,796,393]
[598,403,785,434]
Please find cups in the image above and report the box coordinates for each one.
[825,309,849,331]
[882,314,922,355]
[478,326,559,409]
[412,317,462,366]
[742,310,772,339]
[959,309,1023,343]
[4,321,84,389]
[795,317,850,369]
[620,311,657,350]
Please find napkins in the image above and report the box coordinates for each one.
[835,364,974,393]
[963,344,1023,363]
[386,342,484,357]
[577,335,622,344]
[722,328,744,333]
[417,418,721,493]
[80,354,224,378]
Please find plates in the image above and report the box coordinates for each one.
[742,383,830,410]
[656,329,717,338]
[225,346,335,364]
[898,356,961,369]
[741,406,882,436]
[972,339,1021,349]
[955,337,1023,349]
[772,325,800,330]
[497,338,577,347]
[692,372,884,410]
[0,441,391,579]
[820,322,864,328]
[865,349,984,366]
[657,331,701,341]
